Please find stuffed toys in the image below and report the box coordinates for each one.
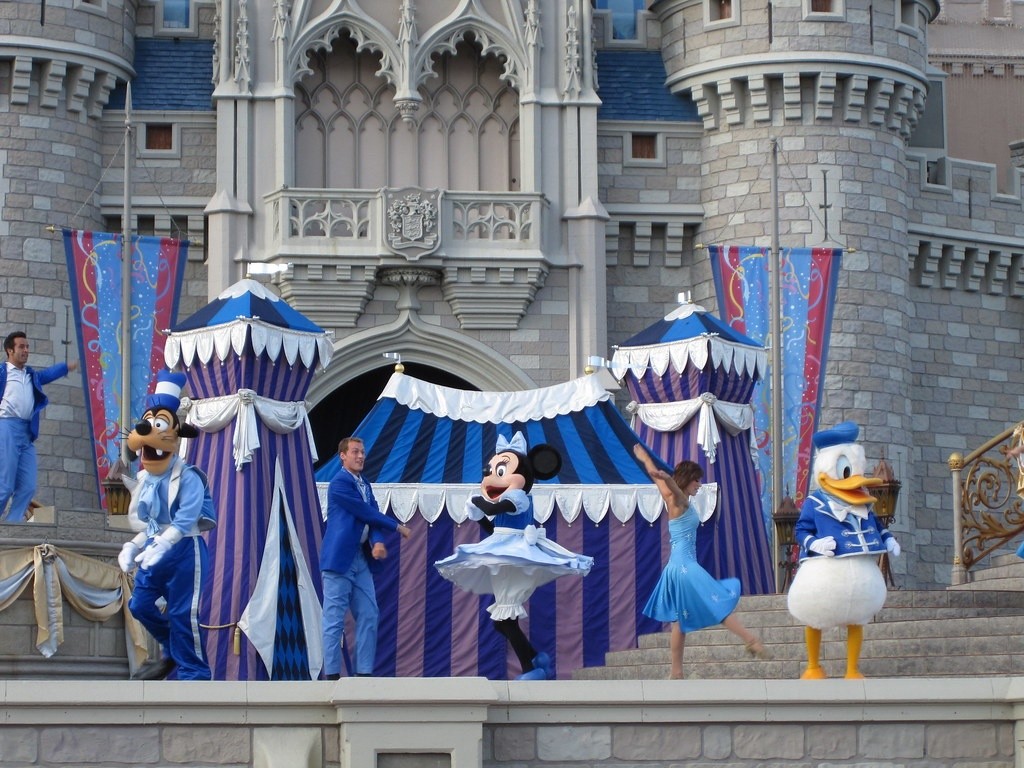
[433,429,595,680]
[118,371,211,680]
[788,421,903,680]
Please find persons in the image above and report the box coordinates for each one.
[319,436,412,680]
[633,442,775,680]
[0,331,77,521]
[1010,423,1024,560]
